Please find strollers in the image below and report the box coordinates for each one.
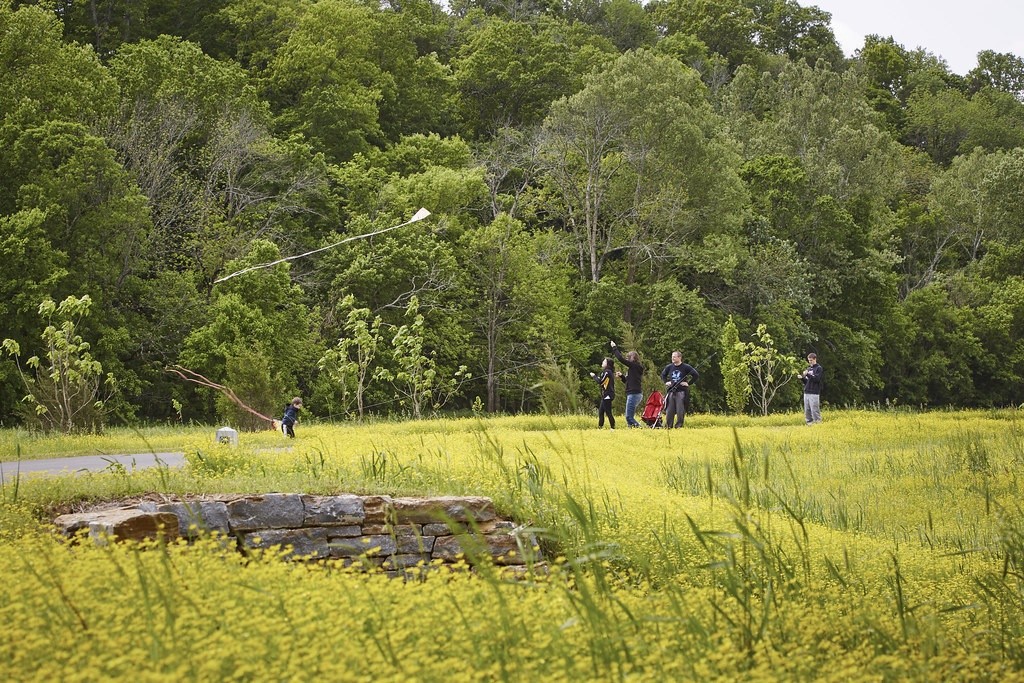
[638,388,670,430]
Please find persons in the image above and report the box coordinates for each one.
[610,340,644,429]
[589,357,616,430]
[661,351,699,430]
[281,396,303,440]
[797,353,823,426]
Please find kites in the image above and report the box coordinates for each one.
[165,365,282,432]
[213,206,431,284]
